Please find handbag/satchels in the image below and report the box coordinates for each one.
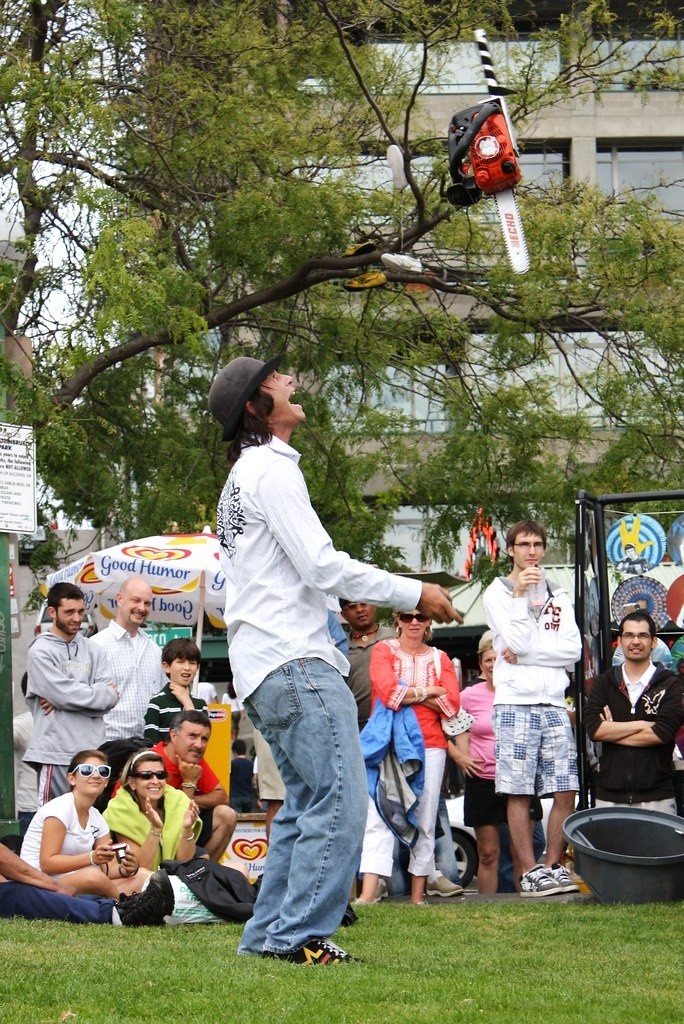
[440,706,474,738]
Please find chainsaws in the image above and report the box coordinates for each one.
[439,29,531,275]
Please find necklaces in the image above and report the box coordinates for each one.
[352,626,378,641]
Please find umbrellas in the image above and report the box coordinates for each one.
[43,525,342,698]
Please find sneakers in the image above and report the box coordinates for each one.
[546,864,580,893]
[264,934,367,969]
[519,866,561,898]
[115,868,175,926]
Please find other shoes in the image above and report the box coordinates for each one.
[425,872,463,898]
[375,878,387,900]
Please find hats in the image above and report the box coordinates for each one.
[209,352,282,440]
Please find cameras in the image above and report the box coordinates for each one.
[109,843,130,864]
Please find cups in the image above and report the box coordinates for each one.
[527,566,547,606]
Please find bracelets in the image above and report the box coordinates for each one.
[150,829,161,837]
[182,783,200,792]
[411,687,418,703]
[180,832,194,841]
[127,862,139,877]
[416,687,427,702]
[90,851,94,864]
[119,865,129,878]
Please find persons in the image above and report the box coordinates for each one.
[482,521,583,896]
[584,610,684,814]
[0,577,548,930]
[207,355,464,964]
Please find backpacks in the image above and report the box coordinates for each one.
[92,736,153,814]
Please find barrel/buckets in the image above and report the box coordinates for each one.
[562,806,684,905]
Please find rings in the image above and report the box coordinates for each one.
[146,811,149,814]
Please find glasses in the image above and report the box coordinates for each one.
[130,770,168,780]
[511,542,544,551]
[71,764,111,779]
[343,601,370,610]
[621,633,652,641]
[399,612,430,623]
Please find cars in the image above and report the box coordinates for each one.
[441,788,589,886]
